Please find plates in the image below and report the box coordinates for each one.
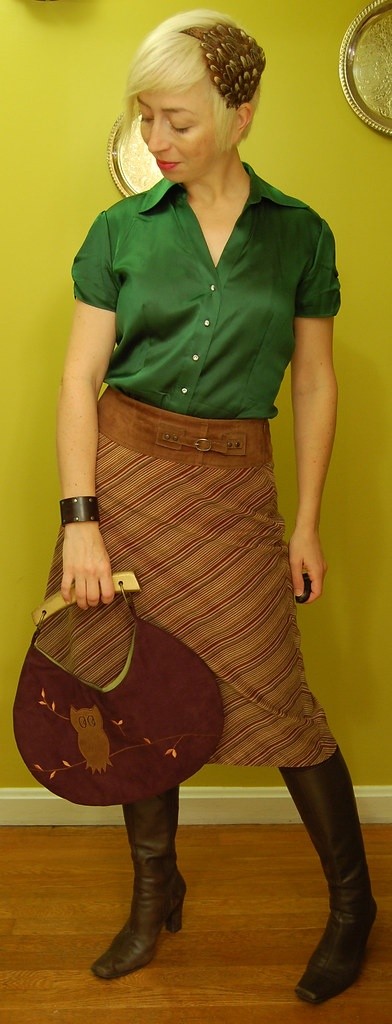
[338,0,392,138]
[107,105,165,198]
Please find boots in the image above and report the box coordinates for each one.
[91,779,186,979]
[277,745,377,1004]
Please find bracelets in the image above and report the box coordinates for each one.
[61,496,100,527]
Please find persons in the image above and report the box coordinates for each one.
[37,11,378,1005]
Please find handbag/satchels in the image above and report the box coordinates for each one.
[13,570,222,807]
